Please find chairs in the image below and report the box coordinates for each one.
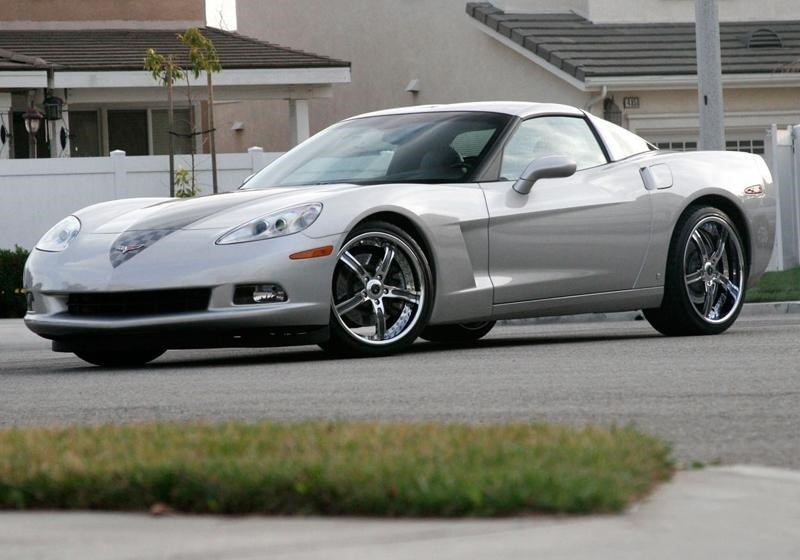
[420,146,467,179]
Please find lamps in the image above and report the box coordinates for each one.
[22,101,44,134]
[43,89,63,121]
[603,96,622,126]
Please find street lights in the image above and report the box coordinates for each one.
[21,100,46,159]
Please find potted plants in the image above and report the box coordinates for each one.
[0,243,32,318]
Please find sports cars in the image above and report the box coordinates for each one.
[20,101,779,370]
